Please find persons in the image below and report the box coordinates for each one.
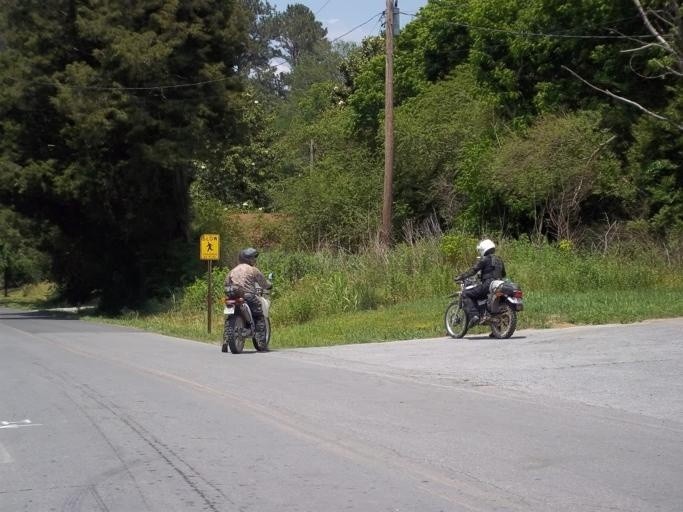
[453,238,506,338]
[222,247,273,352]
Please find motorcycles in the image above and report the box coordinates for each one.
[222,270,276,356]
[442,273,527,340]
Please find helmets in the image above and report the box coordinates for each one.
[476,239,495,258]
[238,248,258,266]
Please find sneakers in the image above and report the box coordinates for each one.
[467,315,479,328]
